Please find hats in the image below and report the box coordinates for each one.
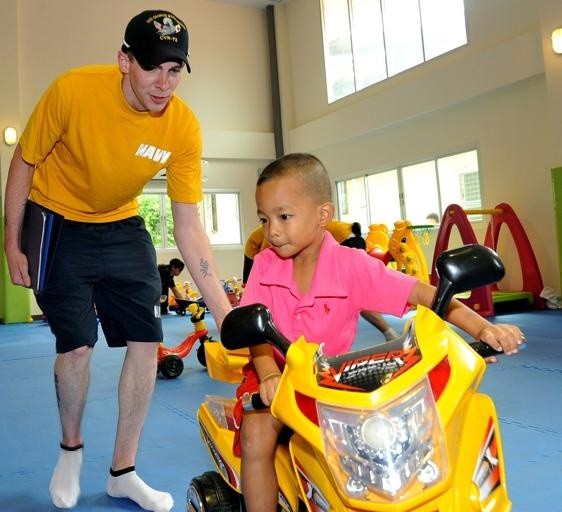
[121,10,192,74]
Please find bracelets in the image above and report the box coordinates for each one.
[261,373,281,382]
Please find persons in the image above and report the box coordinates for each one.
[4,9,234,511]
[157,258,184,316]
[242,224,271,284]
[324,220,366,250]
[240,153,525,512]
